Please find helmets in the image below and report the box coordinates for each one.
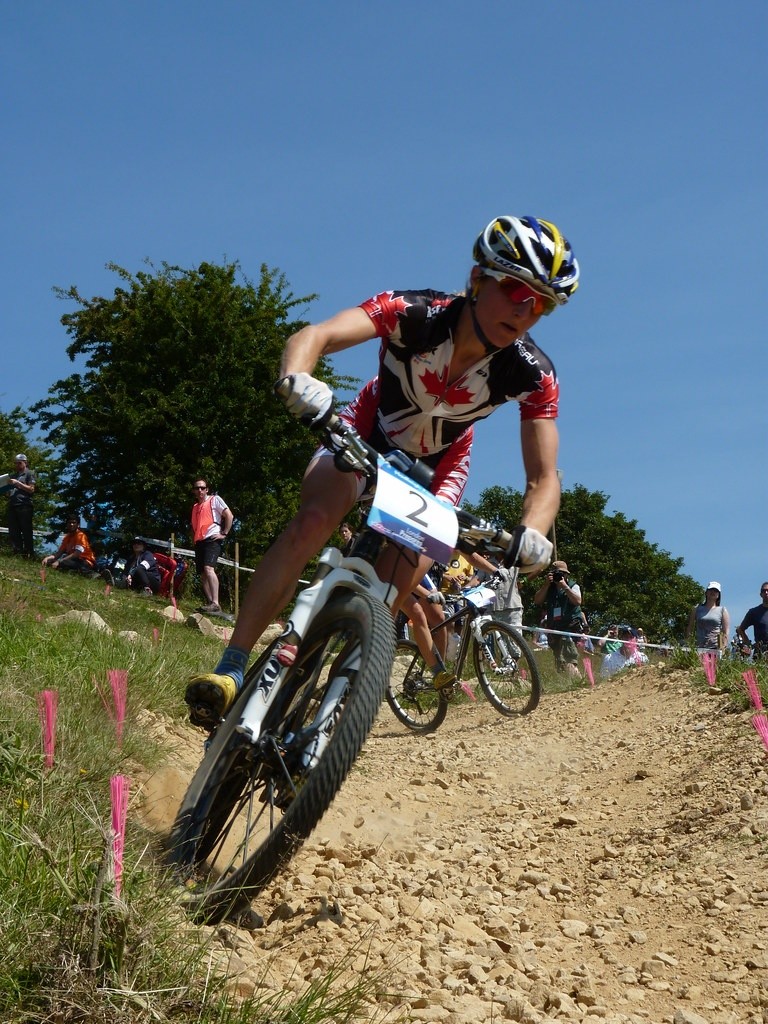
[472,215,580,305]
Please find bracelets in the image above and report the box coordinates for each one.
[221,531,227,536]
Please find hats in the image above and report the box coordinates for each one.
[131,537,147,544]
[552,560,571,575]
[708,581,721,592]
[621,626,639,637]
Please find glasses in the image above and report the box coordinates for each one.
[194,487,206,490]
[132,541,143,545]
[761,589,768,593]
[482,267,557,316]
[14,462,22,465]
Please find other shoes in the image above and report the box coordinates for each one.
[91,572,101,580]
[104,569,115,586]
[143,587,153,596]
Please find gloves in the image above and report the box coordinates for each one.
[493,569,509,582]
[426,591,446,605]
[504,525,554,573]
[287,371,336,433]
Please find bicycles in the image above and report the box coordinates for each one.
[387,572,542,735]
[161,375,551,928]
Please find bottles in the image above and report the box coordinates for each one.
[445,633,461,661]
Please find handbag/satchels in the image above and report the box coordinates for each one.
[223,516,242,539]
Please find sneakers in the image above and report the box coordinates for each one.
[183,674,237,732]
[274,763,313,807]
[200,602,221,612]
[433,671,456,690]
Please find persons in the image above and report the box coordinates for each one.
[191,478,233,613]
[598,624,628,676]
[461,554,523,679]
[103,535,161,598]
[535,560,581,682]
[400,539,508,690]
[737,582,768,659]
[340,521,356,556]
[620,625,642,666]
[183,215,579,809]
[441,550,473,669]
[636,627,647,652]
[6,454,36,558]
[41,515,95,573]
[684,581,729,654]
